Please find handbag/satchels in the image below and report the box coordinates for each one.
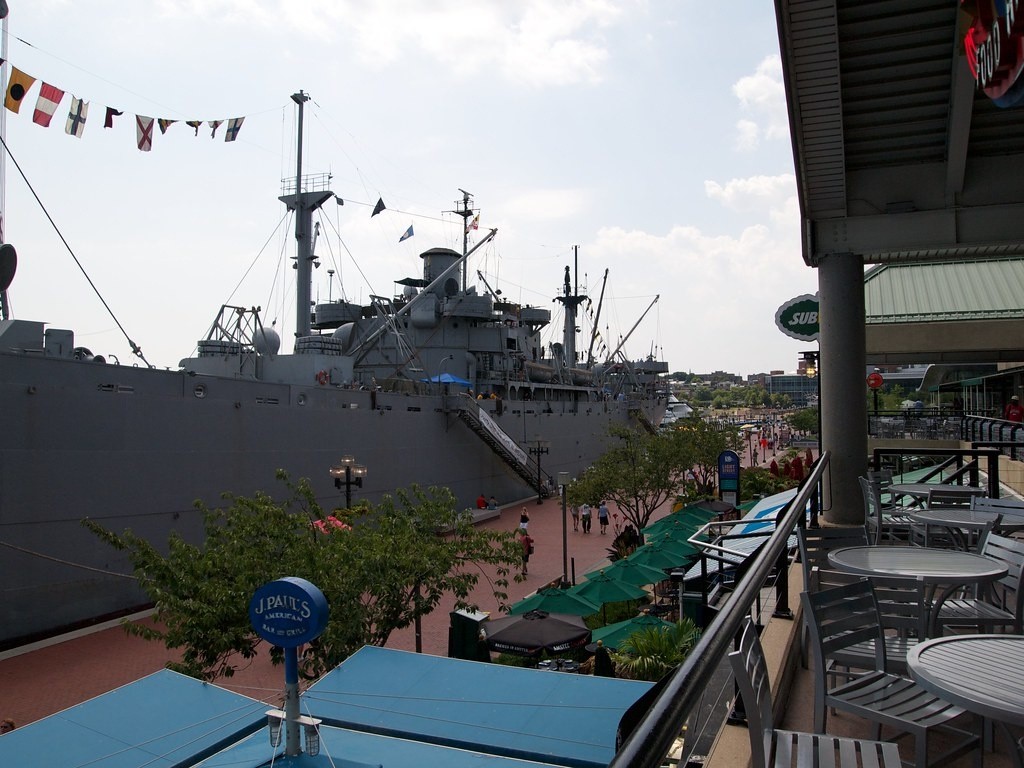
[529,547,534,554]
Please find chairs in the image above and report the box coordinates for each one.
[881,419,960,440]
[728,469,1024,768]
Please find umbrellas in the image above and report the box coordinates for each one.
[420,372,473,387]
[770,448,813,481]
[479,498,759,679]
[309,515,352,533]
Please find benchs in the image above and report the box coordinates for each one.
[523,574,565,600]
[434,508,501,533]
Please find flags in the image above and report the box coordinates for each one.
[159,117,244,142]
[4,66,154,151]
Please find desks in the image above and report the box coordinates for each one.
[888,480,986,510]
[827,545,1009,643]
[909,508,1024,552]
[657,589,679,604]
[535,659,579,672]
[638,604,674,622]
[905,634,1024,768]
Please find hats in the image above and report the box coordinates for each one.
[1011,395,1020,401]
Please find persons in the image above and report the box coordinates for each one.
[549,476,563,495]
[752,425,778,467]
[519,529,534,575]
[597,501,611,535]
[569,502,580,532]
[484,497,499,510]
[476,494,489,509]
[580,503,592,534]
[595,392,624,401]
[686,469,695,487]
[913,394,1024,423]
[519,506,529,528]
[0,718,16,735]
[468,389,498,399]
